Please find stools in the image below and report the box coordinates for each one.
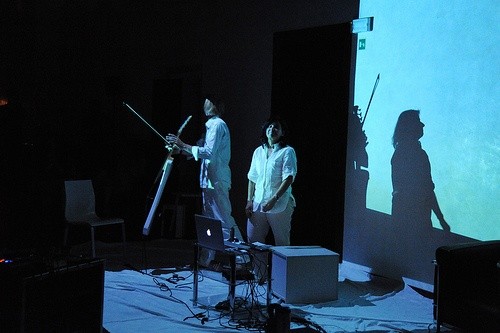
[161,204,192,238]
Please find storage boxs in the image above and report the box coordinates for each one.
[269,245,340,304]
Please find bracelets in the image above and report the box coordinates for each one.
[274,195,279,200]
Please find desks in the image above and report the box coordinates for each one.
[193,243,273,321]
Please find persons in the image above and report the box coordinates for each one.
[245,116,297,284]
[166,96,245,270]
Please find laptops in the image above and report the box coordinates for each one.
[195,214,242,252]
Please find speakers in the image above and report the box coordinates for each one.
[0,252,105,333]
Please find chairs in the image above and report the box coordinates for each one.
[62,179,126,260]
